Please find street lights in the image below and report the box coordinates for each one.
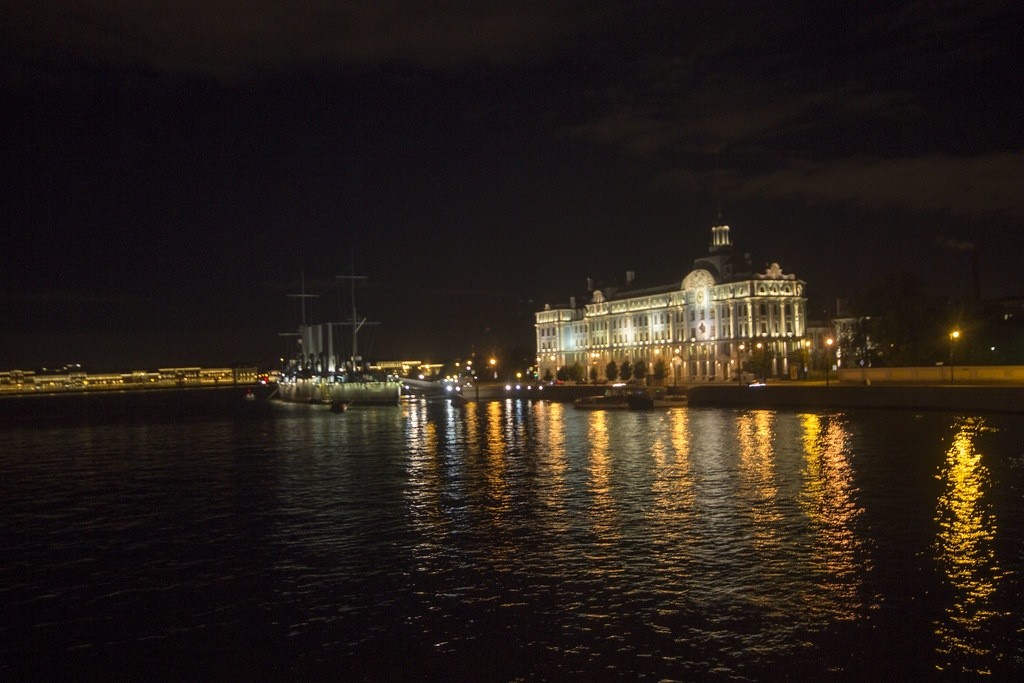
[949,330,959,384]
[824,339,833,385]
[591,353,600,385]
[673,349,680,386]
[803,341,811,380]
[757,343,766,382]
[737,345,744,386]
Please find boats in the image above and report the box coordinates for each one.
[574,385,688,409]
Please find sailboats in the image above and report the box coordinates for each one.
[276,247,402,405]
